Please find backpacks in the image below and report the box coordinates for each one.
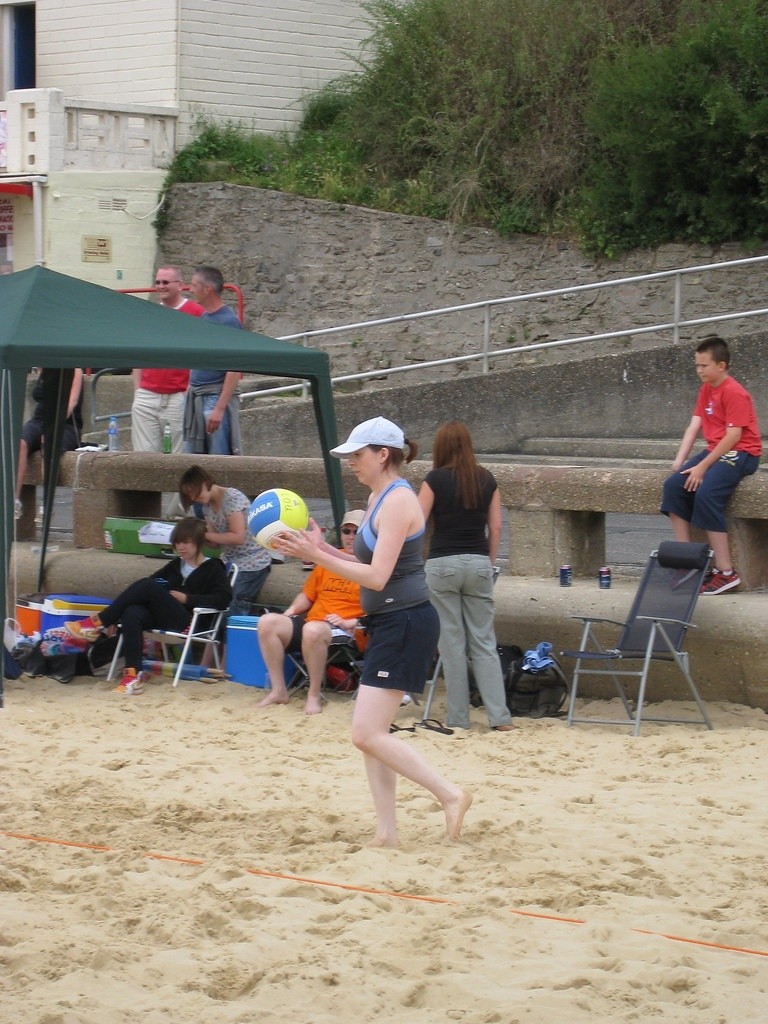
[466,644,569,718]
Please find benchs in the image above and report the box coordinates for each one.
[15,448,768,593]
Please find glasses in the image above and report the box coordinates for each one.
[154,280,180,285]
[341,528,357,534]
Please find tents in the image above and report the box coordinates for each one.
[1,265,347,679]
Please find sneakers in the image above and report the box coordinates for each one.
[15,499,23,519]
[669,568,698,591]
[34,501,55,522]
[112,667,144,694]
[63,613,103,642]
[700,565,741,596]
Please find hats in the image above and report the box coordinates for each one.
[338,509,366,528]
[329,417,404,459]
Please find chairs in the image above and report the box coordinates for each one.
[260,566,500,721]
[559,541,717,736]
[107,560,238,688]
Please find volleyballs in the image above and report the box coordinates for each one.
[245,488,310,551]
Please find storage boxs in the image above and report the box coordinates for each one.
[16,593,112,638]
[104,515,223,558]
[226,615,295,688]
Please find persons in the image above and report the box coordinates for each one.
[14,367,83,523]
[178,464,272,673]
[251,509,369,715]
[64,517,233,695]
[417,421,521,732]
[660,337,762,595]
[131,263,243,519]
[271,417,474,848]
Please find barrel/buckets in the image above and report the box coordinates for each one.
[224,615,295,690]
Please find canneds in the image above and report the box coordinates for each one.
[559,564,572,587]
[599,567,611,588]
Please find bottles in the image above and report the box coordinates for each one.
[163,423,172,453]
[265,671,272,691]
[109,416,118,452]
[321,527,326,539]
[16,634,27,645]
